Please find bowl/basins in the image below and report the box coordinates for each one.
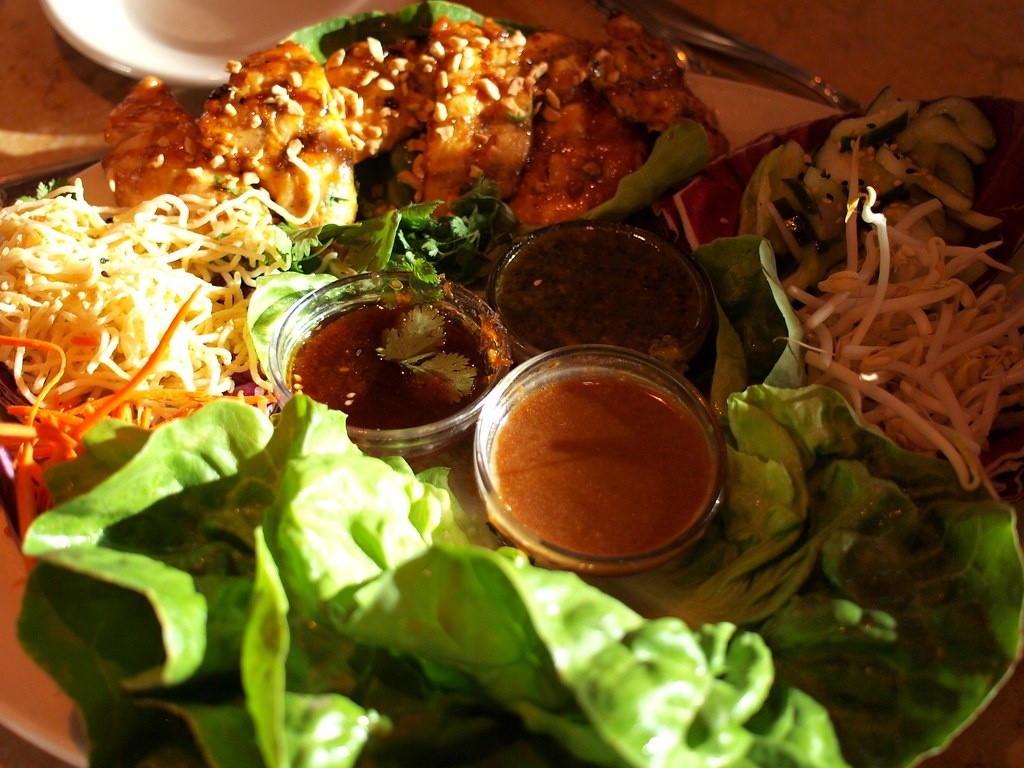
[485,220,715,374]
[472,342,727,580]
[267,268,510,467]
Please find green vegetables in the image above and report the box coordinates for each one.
[248,0,714,407]
[14,233,1024,768]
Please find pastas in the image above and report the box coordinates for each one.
[0,149,322,430]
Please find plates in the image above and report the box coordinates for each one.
[0,71,1024,768]
[38,0,418,90]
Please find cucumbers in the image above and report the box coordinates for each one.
[755,84,1003,301]
